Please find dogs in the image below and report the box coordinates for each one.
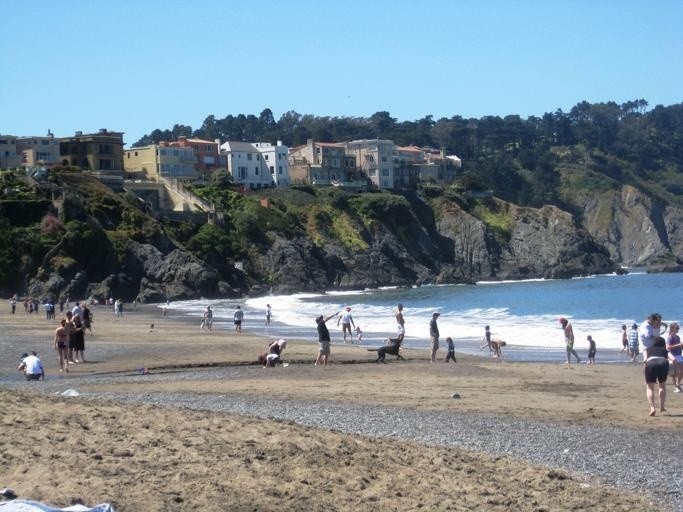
[367,338,405,361]
